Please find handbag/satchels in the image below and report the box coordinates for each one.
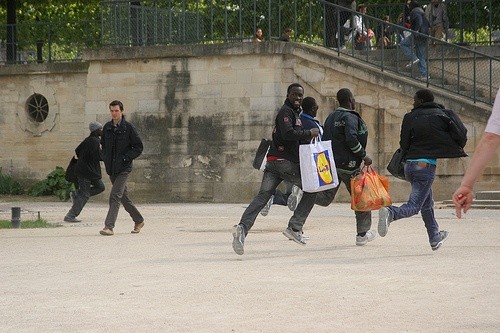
[299,135,339,193]
[387,147,405,180]
[350,165,392,211]
[344,27,356,36]
[253,138,272,172]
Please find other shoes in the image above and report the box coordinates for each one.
[100,226,114,235]
[70,191,76,204]
[416,76,431,80]
[260,195,274,216]
[64,216,79,222]
[407,59,419,68]
[289,235,311,240]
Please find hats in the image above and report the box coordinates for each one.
[89,122,102,133]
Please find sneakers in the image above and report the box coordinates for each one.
[287,185,301,211]
[282,228,306,246]
[356,229,377,246]
[378,207,390,237]
[232,225,244,255]
[431,230,449,251]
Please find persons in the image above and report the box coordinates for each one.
[63,121,106,222]
[377,88,470,251]
[277,28,292,42]
[335,0,450,80]
[232,83,320,256]
[282,88,376,247]
[259,96,324,216]
[99,100,145,236]
[252,28,263,42]
[451,88,500,219]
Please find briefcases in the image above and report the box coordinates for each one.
[131,222,144,233]
